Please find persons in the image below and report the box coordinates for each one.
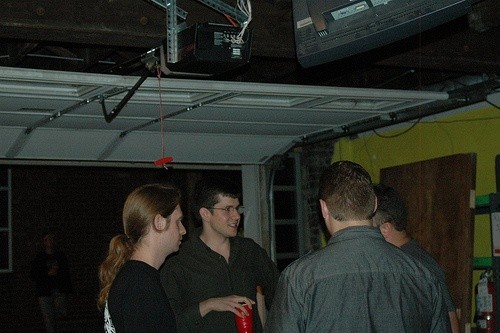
[160,183,276,333]
[27,234,68,333]
[263,161,449,333]
[96,183,186,333]
[373,183,460,333]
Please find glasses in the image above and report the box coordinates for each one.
[211,205,247,215]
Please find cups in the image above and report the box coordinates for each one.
[234,304,253,333]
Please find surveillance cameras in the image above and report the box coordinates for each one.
[141,48,161,63]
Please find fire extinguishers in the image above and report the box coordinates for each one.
[475,268,496,333]
